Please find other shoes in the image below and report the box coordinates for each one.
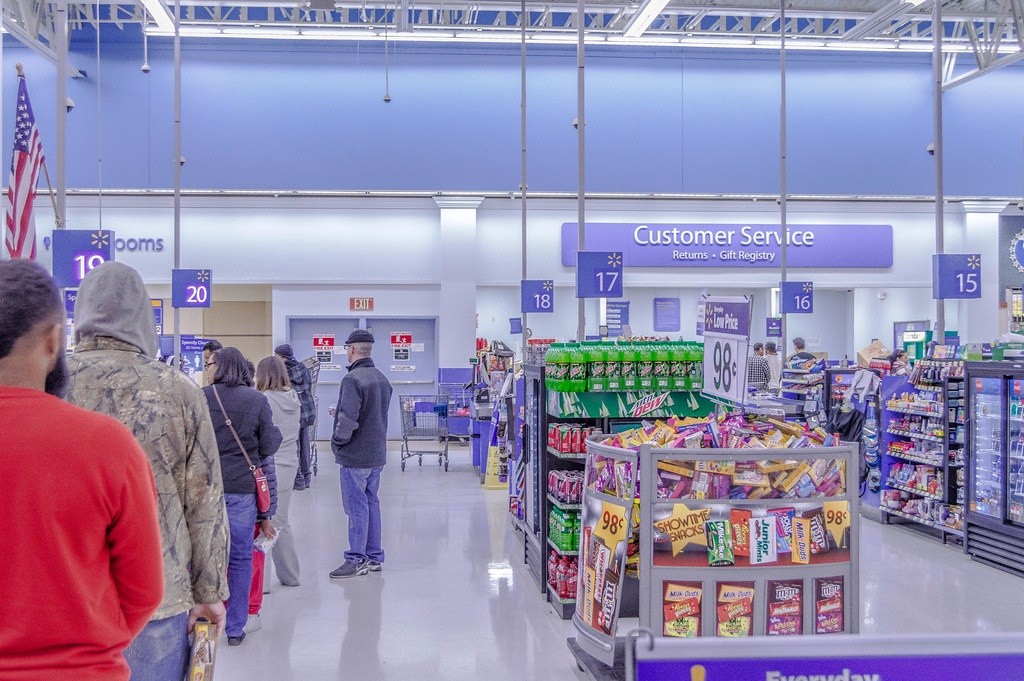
[227,630,245,646]
[242,614,262,633]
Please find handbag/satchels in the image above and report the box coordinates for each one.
[253,468,271,512]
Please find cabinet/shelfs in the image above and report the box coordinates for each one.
[545,445,585,609]
[879,371,963,543]
[781,368,828,421]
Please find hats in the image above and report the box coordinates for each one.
[274,344,293,358]
[345,330,375,343]
[764,341,776,353]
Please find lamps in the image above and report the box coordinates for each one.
[140,0,1024,54]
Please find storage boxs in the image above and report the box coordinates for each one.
[856,340,891,369]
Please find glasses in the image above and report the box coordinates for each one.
[204,362,215,371]
[344,345,354,350]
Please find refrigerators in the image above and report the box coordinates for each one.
[963,360,1024,579]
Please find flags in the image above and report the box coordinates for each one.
[5,75,46,259]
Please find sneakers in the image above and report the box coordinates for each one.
[329,561,369,578]
[367,560,382,571]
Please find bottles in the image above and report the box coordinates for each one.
[544,340,705,392]
[976,397,1024,524]
[548,506,582,600]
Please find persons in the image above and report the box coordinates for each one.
[329,329,393,579]
[62,261,232,681]
[747,338,817,390]
[0,261,165,680]
[200,340,316,646]
[888,349,911,376]
